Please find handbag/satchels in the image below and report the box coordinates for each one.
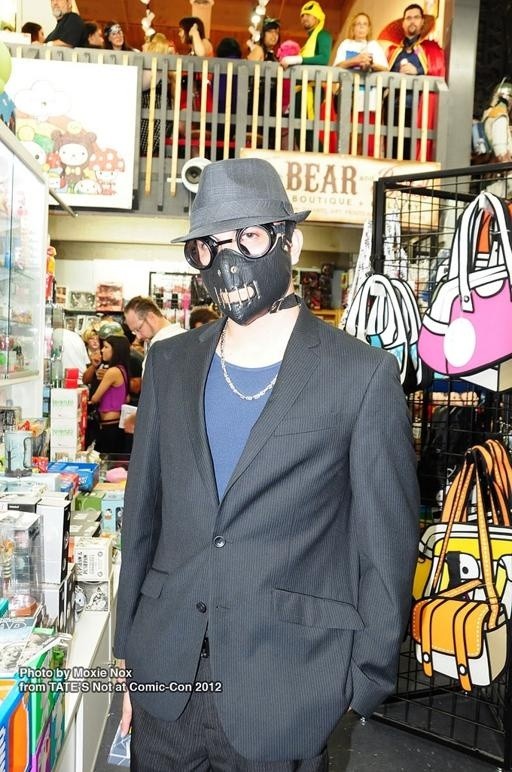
[414,440,510,692]
[345,191,512,378]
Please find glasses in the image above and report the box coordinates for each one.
[184,222,286,270]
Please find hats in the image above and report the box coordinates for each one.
[172,157,311,246]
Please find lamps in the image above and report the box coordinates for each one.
[245,0,269,52]
[139,0,157,39]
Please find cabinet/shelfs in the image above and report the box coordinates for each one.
[1,121,56,387]
[48,557,127,772]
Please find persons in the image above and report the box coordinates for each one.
[480,82,512,201]
[50,294,220,472]
[92,154,421,772]
[1,0,446,161]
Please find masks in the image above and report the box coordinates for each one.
[198,235,290,324]
